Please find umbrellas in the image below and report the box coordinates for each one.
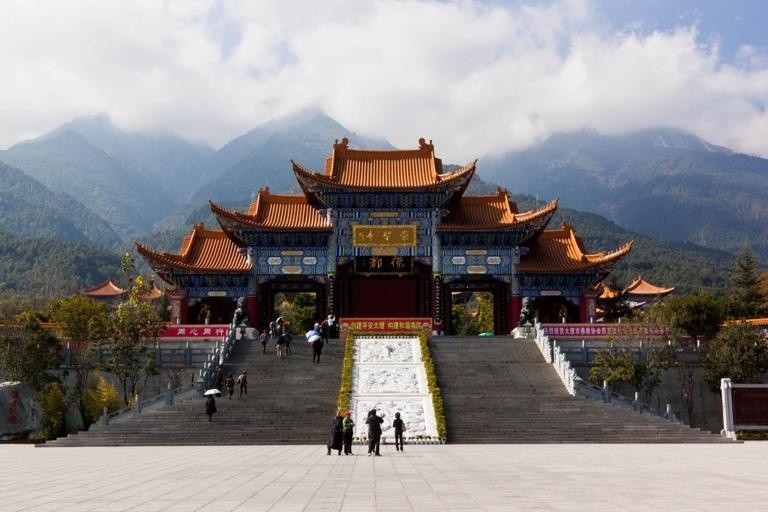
[204,388,222,396]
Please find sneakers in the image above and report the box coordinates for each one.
[367,453,371,456]
[344,453,353,456]
[327,453,331,455]
[396,448,403,452]
[338,453,341,456]
[373,450,382,456]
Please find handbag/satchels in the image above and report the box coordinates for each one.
[402,422,406,432]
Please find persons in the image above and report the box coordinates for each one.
[206,395,217,421]
[327,410,405,455]
[215,314,336,398]
